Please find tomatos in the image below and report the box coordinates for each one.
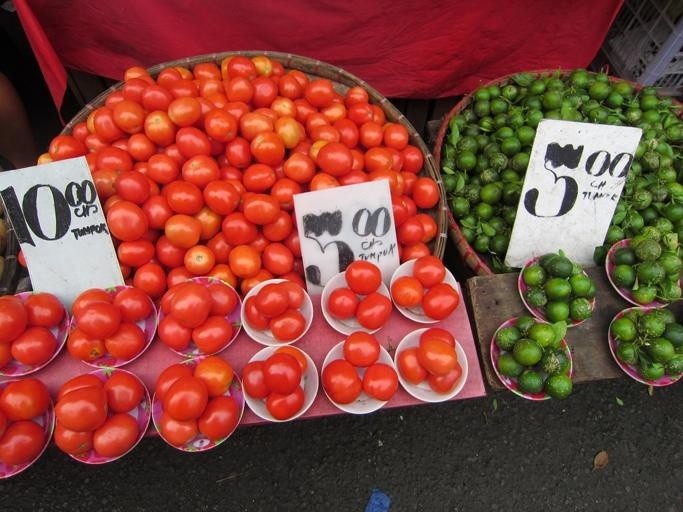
[328,261,393,331]
[245,282,307,340]
[306,71,440,258]
[398,329,461,395]
[66,290,152,362]
[158,284,240,355]
[0,377,53,464]
[53,374,146,456]
[18,56,305,293]
[322,331,399,405]
[0,293,66,369]
[242,348,309,419]
[394,257,458,320]
[156,357,240,446]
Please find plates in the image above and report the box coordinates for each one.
[240,279,315,344]
[154,353,245,454]
[394,328,473,402]
[56,367,153,465]
[157,277,244,357]
[4,291,69,377]
[321,339,397,415]
[606,308,682,388]
[242,346,319,423]
[392,259,460,324]
[606,236,672,308]
[518,253,596,327]
[489,317,574,402]
[321,272,393,337]
[1,380,55,478]
[69,285,159,368]
